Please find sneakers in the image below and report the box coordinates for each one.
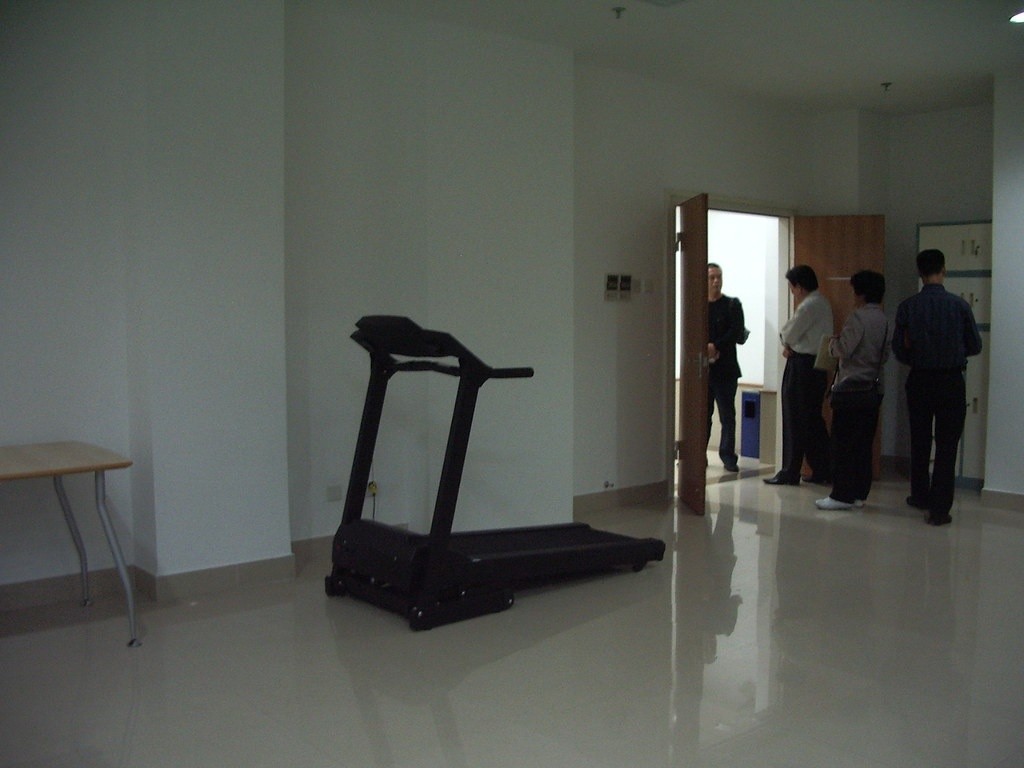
[816,497,852,509]
[856,500,865,507]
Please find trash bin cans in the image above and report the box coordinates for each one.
[741,391,760,459]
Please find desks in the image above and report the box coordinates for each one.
[0,441,144,650]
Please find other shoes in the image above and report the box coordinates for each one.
[928,515,952,525]
[724,464,739,472]
[906,496,927,509]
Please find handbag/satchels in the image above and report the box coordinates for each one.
[826,377,884,409]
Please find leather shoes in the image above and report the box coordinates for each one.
[763,476,800,485]
[802,477,831,483]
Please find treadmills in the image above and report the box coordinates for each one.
[322,311,668,634]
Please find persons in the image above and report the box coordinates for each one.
[815,269,891,510]
[705,263,747,472]
[762,265,834,487]
[890,249,984,524]
